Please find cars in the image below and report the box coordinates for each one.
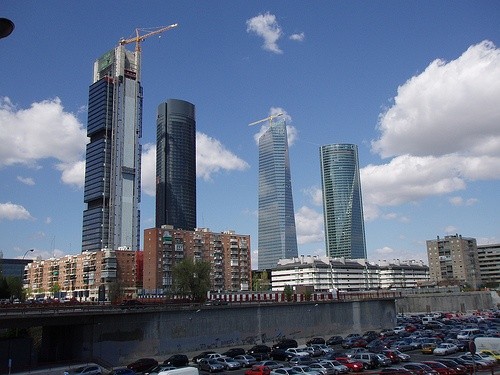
[205,299,228,306]
[343,337,367,350]
[285,348,310,358]
[364,307,500,375]
[121,300,144,309]
[319,360,349,375]
[292,365,321,375]
[304,334,362,362]
[163,354,189,368]
[335,357,363,373]
[70,365,101,375]
[270,368,306,375]
[107,368,141,375]
[127,358,158,373]
[310,363,334,375]
[271,338,299,351]
[289,357,314,368]
[1,297,70,305]
[190,345,287,372]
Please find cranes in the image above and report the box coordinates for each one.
[119,22,179,83]
[248,112,284,129]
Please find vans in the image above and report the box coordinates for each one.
[148,366,198,375]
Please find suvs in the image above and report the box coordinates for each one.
[243,365,271,375]
[352,352,379,370]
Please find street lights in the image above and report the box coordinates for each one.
[20,249,34,281]
